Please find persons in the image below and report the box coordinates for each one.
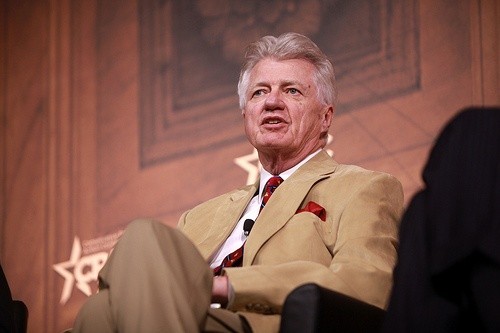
[380,105,499,333]
[73,32,404,333]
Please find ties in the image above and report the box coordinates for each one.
[213,177,284,274]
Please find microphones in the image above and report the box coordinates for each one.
[243,218,257,236]
